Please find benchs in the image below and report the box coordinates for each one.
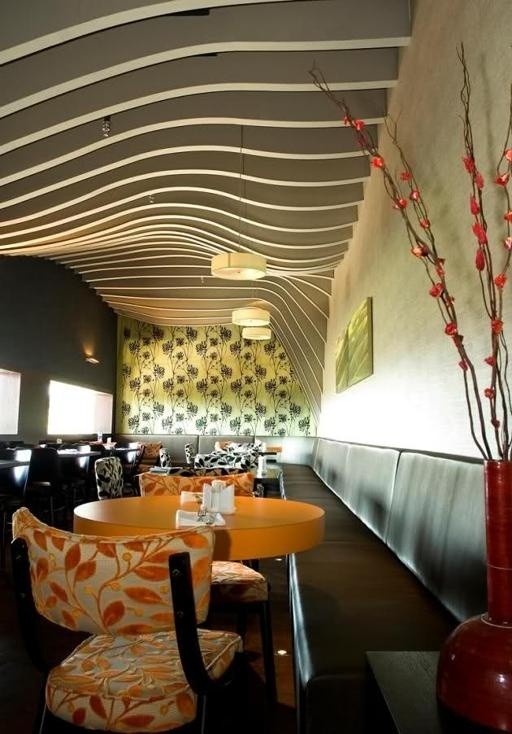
[113,432,484,734]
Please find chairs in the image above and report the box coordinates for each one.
[0,433,323,734]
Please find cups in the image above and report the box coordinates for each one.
[106,436,112,443]
[57,438,62,443]
[211,483,224,513]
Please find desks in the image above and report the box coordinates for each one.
[364,646,452,733]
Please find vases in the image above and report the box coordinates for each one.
[429,457,512,734]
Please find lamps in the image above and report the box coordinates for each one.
[205,123,267,280]
[241,327,272,340]
[230,283,271,326]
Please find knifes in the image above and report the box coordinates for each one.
[205,512,218,526]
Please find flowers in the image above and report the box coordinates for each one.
[303,38,512,461]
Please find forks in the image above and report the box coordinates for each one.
[196,507,207,523]
